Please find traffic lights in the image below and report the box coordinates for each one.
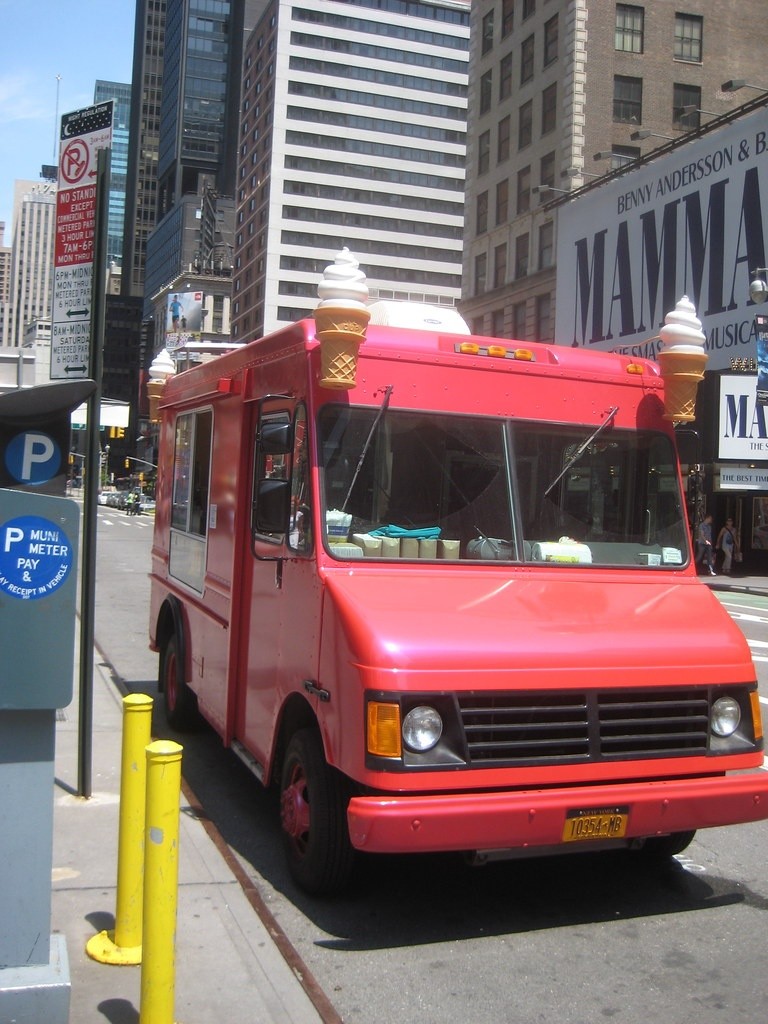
[120,426,125,437]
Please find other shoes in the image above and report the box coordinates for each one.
[709,572,716,576]
[722,569,731,575]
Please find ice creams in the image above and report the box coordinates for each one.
[657,294,708,422]
[312,245,371,390]
[147,348,176,423]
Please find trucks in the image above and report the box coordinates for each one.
[147,247,768,904]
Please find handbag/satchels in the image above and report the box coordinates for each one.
[734,552,742,562]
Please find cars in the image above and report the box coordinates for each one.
[97,491,134,511]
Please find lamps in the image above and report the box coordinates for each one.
[593,150,636,162]
[630,129,675,141]
[560,168,601,178]
[675,104,722,119]
[532,184,568,193]
[721,79,768,92]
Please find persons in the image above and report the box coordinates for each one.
[694,516,741,577]
[127,491,141,516]
[170,295,188,332]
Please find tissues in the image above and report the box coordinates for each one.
[532,536,592,562]
